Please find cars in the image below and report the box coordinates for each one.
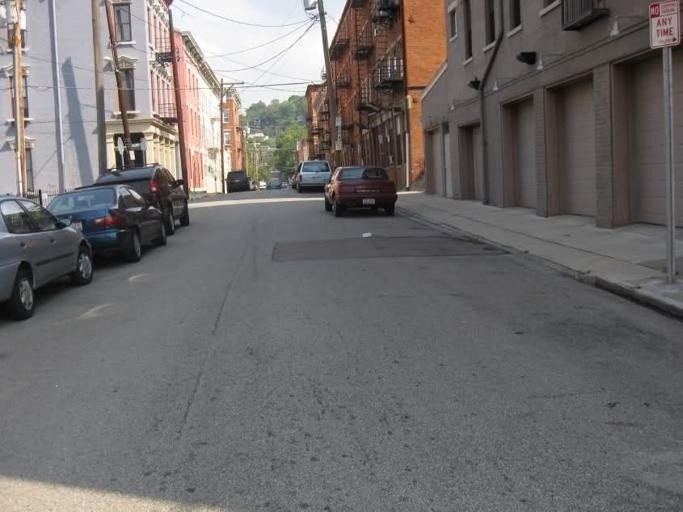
[0,162,190,321]
[225,171,251,192]
[323,164,398,219]
[259,176,284,190]
[290,160,332,193]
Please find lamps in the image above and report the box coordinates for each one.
[450,76,514,112]
[536,14,650,72]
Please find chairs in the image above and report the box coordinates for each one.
[73,194,110,207]
